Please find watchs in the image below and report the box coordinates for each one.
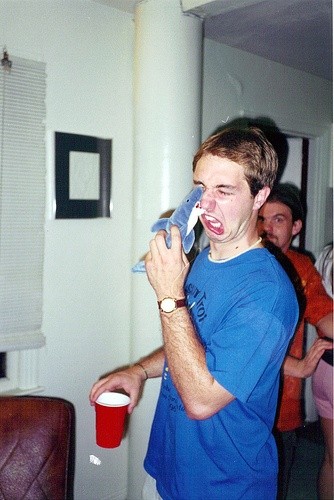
[157,297,186,313]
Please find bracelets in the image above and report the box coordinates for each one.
[134,363,148,379]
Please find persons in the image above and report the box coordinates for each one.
[88,124,301,500]
[256,181,334,500]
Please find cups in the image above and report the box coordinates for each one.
[94,391,131,449]
